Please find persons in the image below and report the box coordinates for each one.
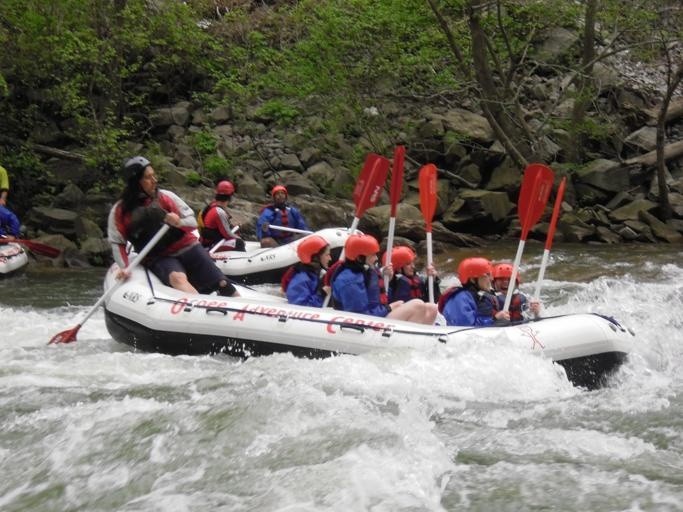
[284,234,333,308]
[494,262,546,321]
[197,179,247,253]
[439,257,511,327]
[380,244,442,305]
[0,202,20,245]
[105,154,242,299]
[0,164,11,206]
[329,233,439,326]
[255,185,313,248]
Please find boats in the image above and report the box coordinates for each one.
[192,228,366,285]
[0,233,30,278]
[103,227,633,388]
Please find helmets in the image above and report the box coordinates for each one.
[494,261,520,286]
[458,257,494,286]
[344,235,380,261]
[271,185,288,199]
[123,155,151,179]
[381,245,417,273]
[297,236,328,264]
[214,180,235,196]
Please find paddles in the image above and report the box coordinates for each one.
[384,145,405,296]
[534,176,567,302]
[502,163,554,312]
[322,152,390,308]
[0,239,61,259]
[418,163,437,303]
[47,224,170,344]
